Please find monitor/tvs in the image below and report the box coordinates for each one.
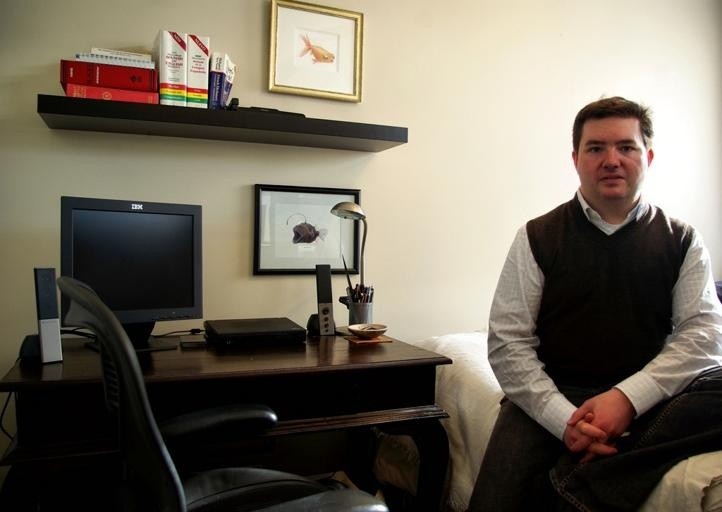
[60,194,202,355]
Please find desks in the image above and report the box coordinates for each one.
[0,330,453,512]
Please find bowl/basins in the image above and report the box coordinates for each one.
[348,323,388,339]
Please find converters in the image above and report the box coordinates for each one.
[178,334,206,351]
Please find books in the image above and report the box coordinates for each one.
[76,52,156,69]
[59,58,159,93]
[159,29,186,108]
[89,46,153,62]
[187,32,210,110]
[67,83,160,106]
[209,51,238,109]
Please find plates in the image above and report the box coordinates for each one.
[344,335,391,345]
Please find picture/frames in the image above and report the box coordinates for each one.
[253,183,361,275]
[268,0,364,103]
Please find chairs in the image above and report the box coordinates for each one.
[57,276,389,512]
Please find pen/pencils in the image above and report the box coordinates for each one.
[346,284,375,304]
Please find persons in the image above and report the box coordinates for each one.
[467,96,721,512]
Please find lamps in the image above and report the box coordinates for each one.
[331,202,367,285]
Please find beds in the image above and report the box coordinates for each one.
[374,330,721,511]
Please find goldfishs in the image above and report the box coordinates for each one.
[299,34,334,64]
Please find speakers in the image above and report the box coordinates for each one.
[32,266,64,365]
[316,263,336,337]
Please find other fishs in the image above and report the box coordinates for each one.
[287,212,327,243]
